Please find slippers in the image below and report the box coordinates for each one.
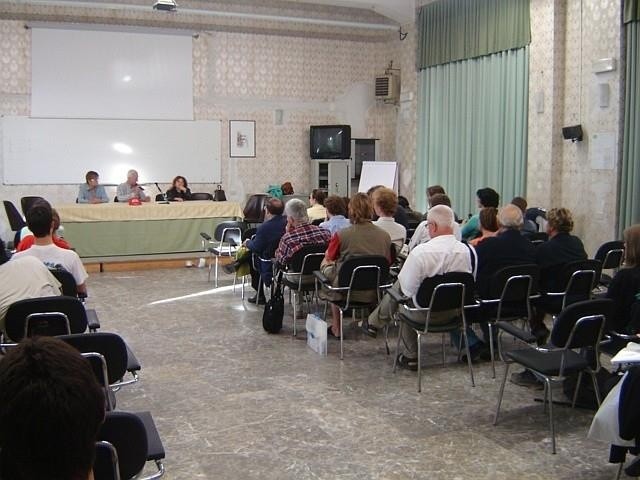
[327,325,340,339]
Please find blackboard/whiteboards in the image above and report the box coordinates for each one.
[0,115,222,184]
[22,19,201,122]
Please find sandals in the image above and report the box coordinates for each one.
[350,318,377,339]
[397,352,420,370]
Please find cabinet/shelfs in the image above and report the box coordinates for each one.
[310,158,351,201]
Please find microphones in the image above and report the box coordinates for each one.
[135,183,144,190]
[155,182,168,203]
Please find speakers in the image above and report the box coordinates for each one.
[376,75,395,101]
[561,125,582,139]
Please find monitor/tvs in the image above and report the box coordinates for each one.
[310,125,351,160]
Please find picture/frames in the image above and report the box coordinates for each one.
[229,120,256,158]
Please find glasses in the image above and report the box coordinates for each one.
[424,223,431,228]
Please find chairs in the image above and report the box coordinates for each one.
[523,232,549,246]
[393,283,481,393]
[156,194,168,201]
[49,269,88,302]
[313,255,394,360]
[608,293,640,386]
[494,298,609,455]
[243,195,268,231]
[252,239,291,307]
[497,270,595,363]
[191,193,212,200]
[92,410,165,480]
[272,245,328,336]
[457,275,532,387]
[233,228,257,300]
[0,296,100,357]
[201,222,244,287]
[593,241,626,303]
[55,332,141,412]
[4,201,27,231]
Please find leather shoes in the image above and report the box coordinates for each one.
[223,262,239,274]
[248,294,266,305]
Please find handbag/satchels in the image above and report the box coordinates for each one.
[531,289,562,346]
[307,311,327,355]
[262,270,285,334]
[213,185,226,201]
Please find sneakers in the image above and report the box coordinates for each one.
[624,455,640,477]
[510,369,544,390]
[289,310,303,319]
[462,341,483,362]
[480,338,492,361]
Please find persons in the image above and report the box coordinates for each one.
[458,188,501,243]
[222,198,289,305]
[423,185,445,220]
[341,196,351,205]
[78,171,109,204]
[357,204,472,371]
[366,185,387,196]
[408,193,462,253]
[1,337,104,480]
[451,204,534,364]
[11,205,87,294]
[16,208,72,250]
[526,208,588,347]
[370,187,407,265]
[588,331,640,479]
[320,193,391,340]
[20,201,64,238]
[275,198,331,319]
[319,195,354,237]
[511,225,640,391]
[428,194,451,208]
[510,197,536,232]
[469,208,500,246]
[397,195,409,208]
[164,176,192,202]
[0,239,62,353]
[307,189,327,224]
[116,169,150,202]
